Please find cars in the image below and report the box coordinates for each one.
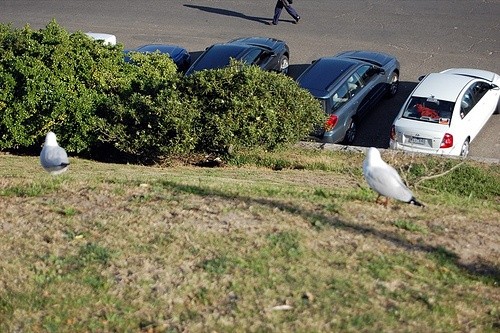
[388,67,500,161]
[181,35,289,87]
[295,48,400,145]
[123,42,192,76]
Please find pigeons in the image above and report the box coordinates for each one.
[41,131,71,176]
[362,147,427,210]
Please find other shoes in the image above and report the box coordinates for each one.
[269,22,277,25]
[296,16,302,23]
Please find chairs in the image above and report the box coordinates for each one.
[347,76,358,90]
[333,93,348,102]
[426,98,440,109]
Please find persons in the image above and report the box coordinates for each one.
[269,0,304,27]
